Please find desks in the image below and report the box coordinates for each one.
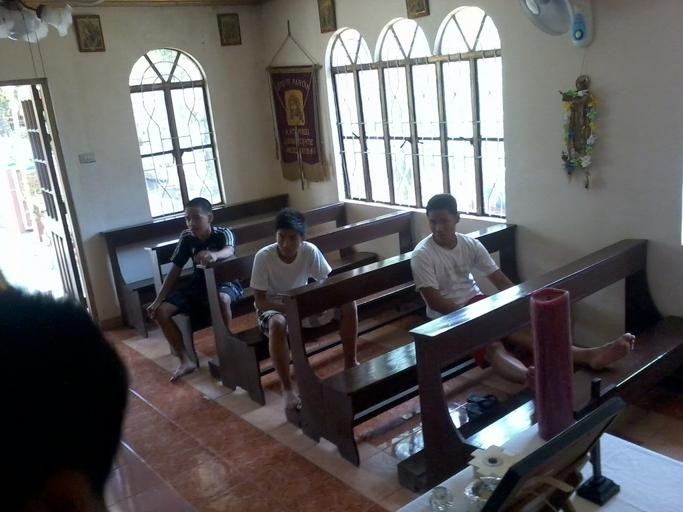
[386,422,682,512]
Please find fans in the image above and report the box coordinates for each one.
[515,0,593,49]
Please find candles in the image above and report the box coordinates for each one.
[525,286,573,437]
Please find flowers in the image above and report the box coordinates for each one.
[554,73,600,193]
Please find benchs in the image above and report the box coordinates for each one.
[279,222,518,466]
[194,211,413,406]
[397,238,681,490]
[142,201,375,365]
[96,195,288,337]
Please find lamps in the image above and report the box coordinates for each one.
[0,0,75,49]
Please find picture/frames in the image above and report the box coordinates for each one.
[317,1,336,33]
[71,13,104,52]
[216,14,241,47]
[405,1,430,20]
[478,395,627,511]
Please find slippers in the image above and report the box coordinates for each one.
[466,403,489,416]
[466,393,500,407]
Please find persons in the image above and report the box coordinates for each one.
[144,197,245,382]
[409,193,637,394]
[249,207,361,410]
[1,284,129,512]
